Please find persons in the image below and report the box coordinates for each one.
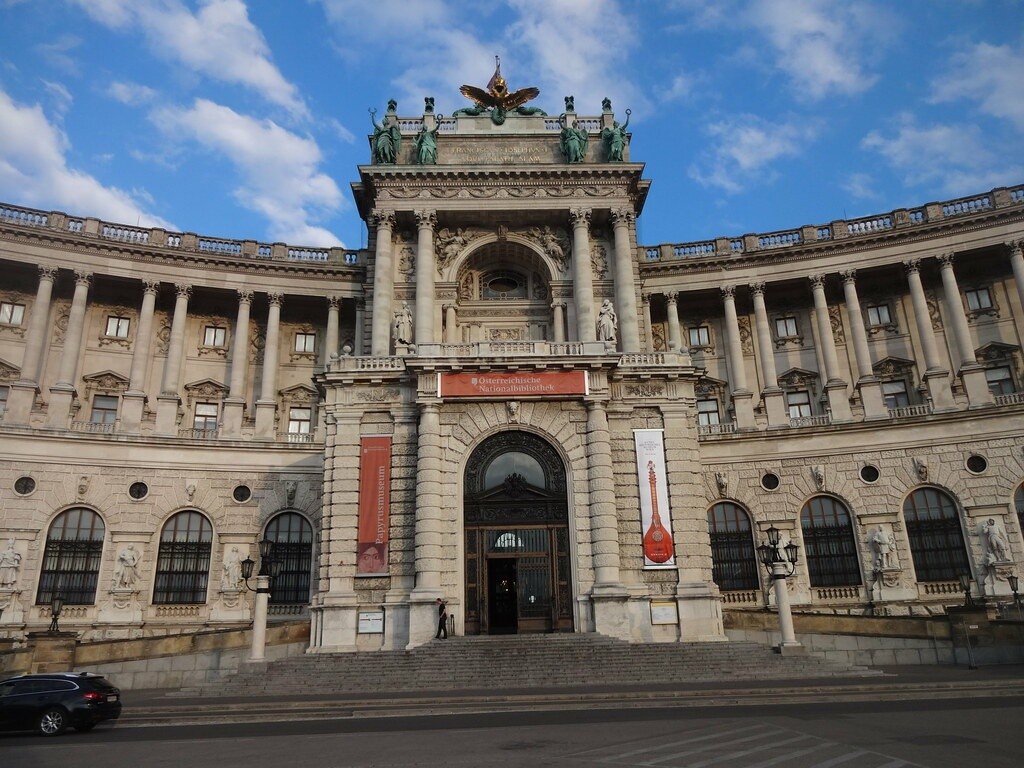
[369,107,401,165]
[396,301,414,345]
[0,536,22,590]
[597,299,618,342]
[115,544,140,588]
[413,120,441,165]
[436,227,470,270]
[357,543,385,573]
[982,517,1011,562]
[558,113,589,164]
[531,225,568,264]
[872,524,894,567]
[434,598,447,641]
[602,108,632,163]
[223,546,243,588]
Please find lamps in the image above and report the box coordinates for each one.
[1006,572,1024,623]
[756,524,801,580]
[240,555,270,593]
[46,587,68,636]
[956,568,978,612]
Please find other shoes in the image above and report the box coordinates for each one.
[434,636,439,638]
[442,637,447,639]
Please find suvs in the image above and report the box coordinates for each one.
[0,671,123,738]
